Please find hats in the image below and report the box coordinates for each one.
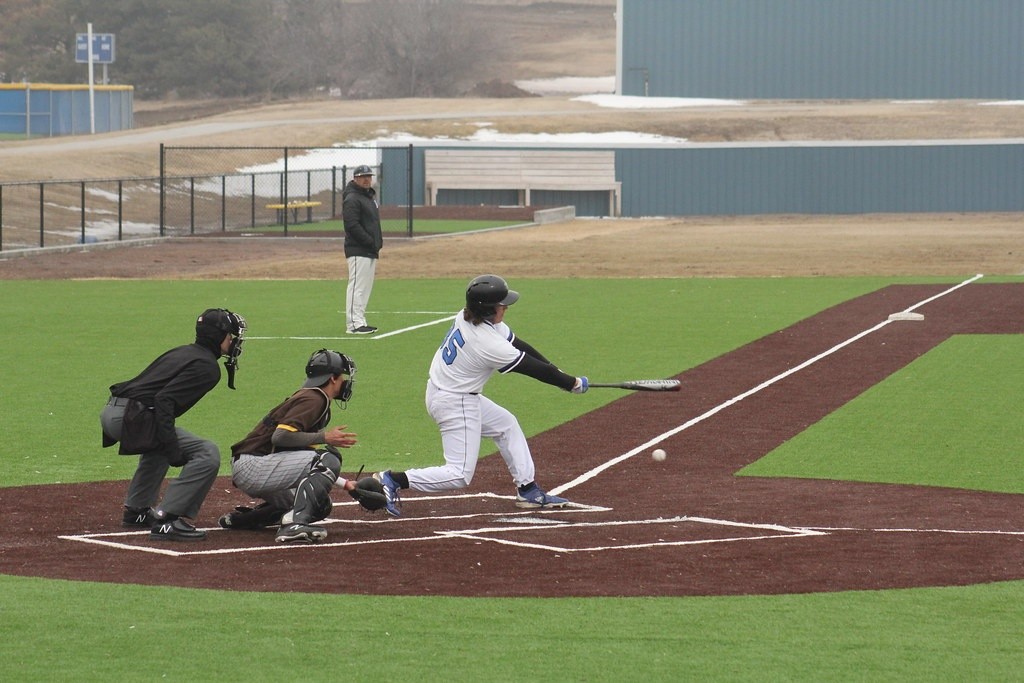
[303,350,350,389]
[353,165,375,176]
[199,309,231,349]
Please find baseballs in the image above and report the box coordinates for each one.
[651,449,666,463]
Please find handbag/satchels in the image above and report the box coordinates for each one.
[119,397,162,457]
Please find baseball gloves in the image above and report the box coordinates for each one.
[354,475,389,512]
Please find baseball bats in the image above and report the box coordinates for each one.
[589,378,682,393]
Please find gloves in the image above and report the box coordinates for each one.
[572,377,589,395]
[168,446,186,467]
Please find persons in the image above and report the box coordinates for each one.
[217,348,359,544]
[101,308,248,540]
[372,275,589,518]
[343,165,383,334]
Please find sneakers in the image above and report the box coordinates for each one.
[347,326,378,334]
[516,485,569,509]
[274,524,327,544]
[122,508,159,526]
[372,470,401,516]
[151,520,203,541]
[218,511,238,528]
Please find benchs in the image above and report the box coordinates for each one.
[265,200,321,225]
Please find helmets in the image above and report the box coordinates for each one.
[466,275,518,306]
